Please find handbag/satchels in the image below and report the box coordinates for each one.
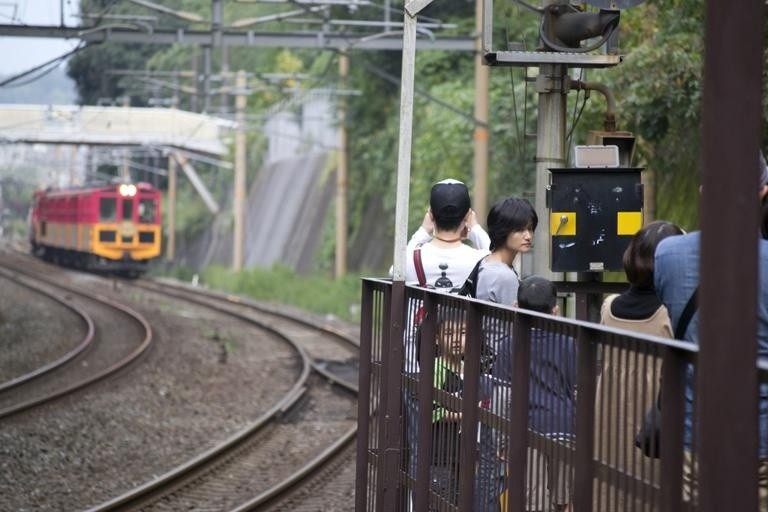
[633,403,663,457]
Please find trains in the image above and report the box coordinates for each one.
[24,180,162,280]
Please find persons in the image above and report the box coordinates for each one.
[433,307,485,510]
[491,274,606,511]
[388,177,493,511]
[594,218,689,512]
[653,147,768,512]
[464,194,537,511]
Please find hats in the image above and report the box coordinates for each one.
[431,178,470,221]
